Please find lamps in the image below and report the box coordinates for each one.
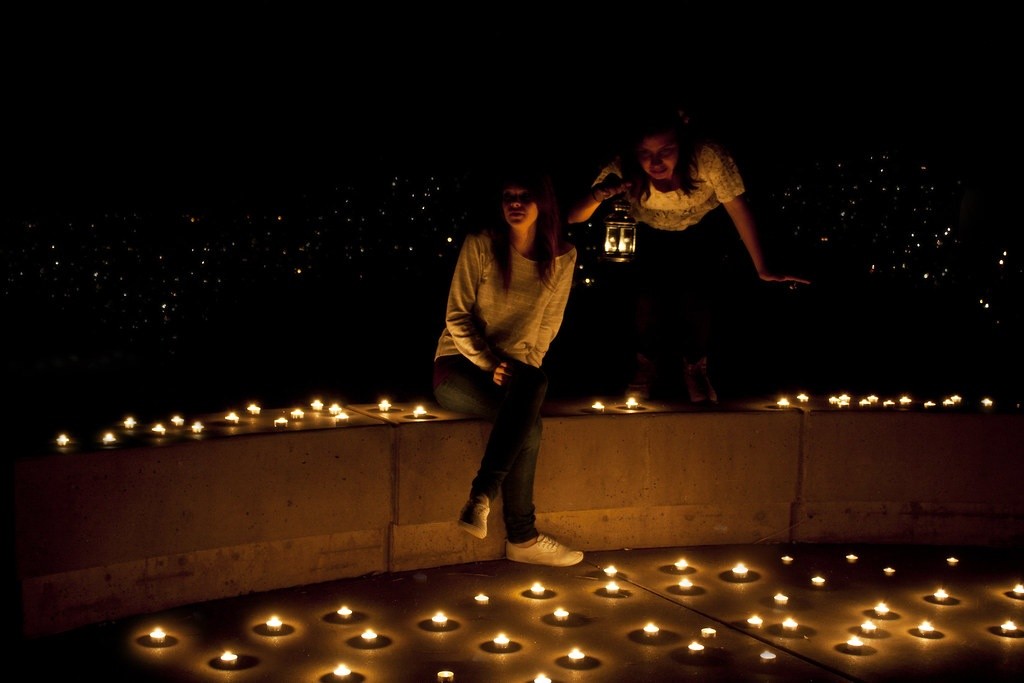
[597,197,638,263]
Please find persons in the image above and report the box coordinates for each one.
[568,97,808,405]
[429,163,587,567]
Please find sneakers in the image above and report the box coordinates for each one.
[458,496,490,540]
[505,533,583,566]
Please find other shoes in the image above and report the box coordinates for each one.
[684,357,717,402]
[625,354,655,397]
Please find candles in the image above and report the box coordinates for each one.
[50,388,1024,682]
